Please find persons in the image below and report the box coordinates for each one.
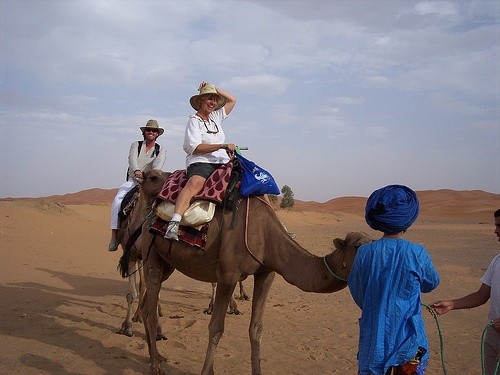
[164,81,297,241]
[347,185,439,375]
[425,209,500,375]
[108,119,168,251]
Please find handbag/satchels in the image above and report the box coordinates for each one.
[234,151,280,198]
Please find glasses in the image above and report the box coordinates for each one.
[146,128,158,132]
[204,120,219,134]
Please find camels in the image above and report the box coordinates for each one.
[116,167,380,375]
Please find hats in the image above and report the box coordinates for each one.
[139,120,164,135]
[190,83,227,111]
[365,185,420,235]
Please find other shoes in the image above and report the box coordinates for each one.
[164,220,179,242]
[109,238,118,251]
